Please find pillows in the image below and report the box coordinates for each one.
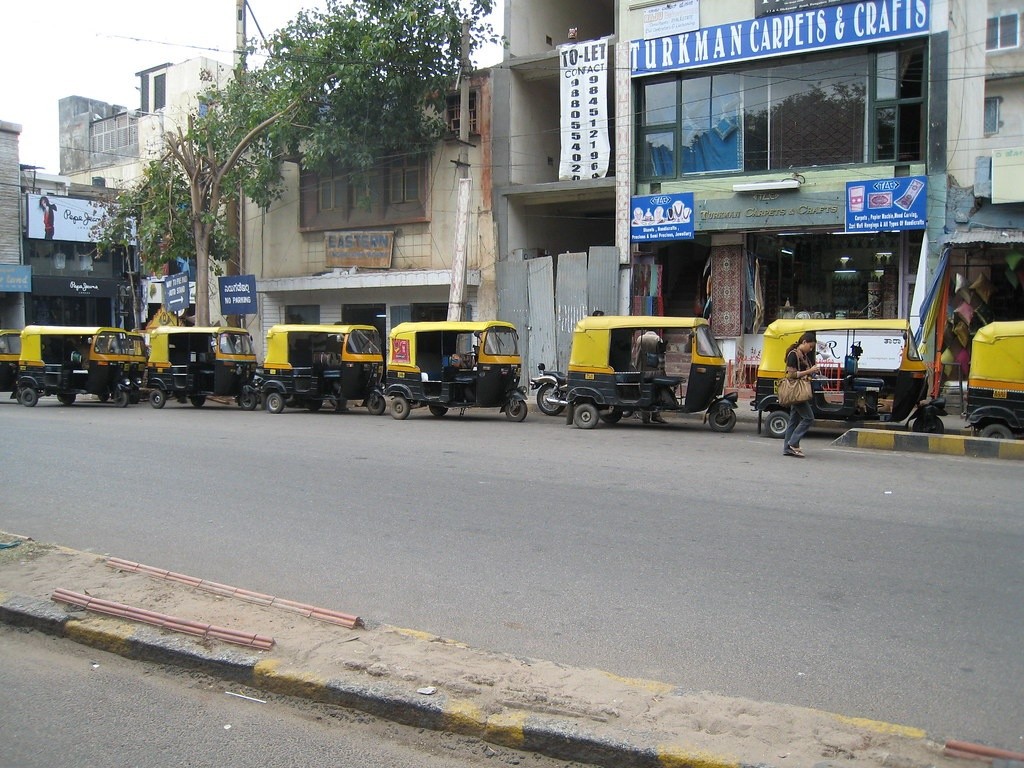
[939,250,1024,378]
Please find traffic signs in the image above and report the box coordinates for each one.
[164,271,189,310]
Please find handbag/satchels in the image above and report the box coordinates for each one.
[777,350,815,407]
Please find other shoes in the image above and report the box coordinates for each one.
[643,419,652,423]
[651,416,667,423]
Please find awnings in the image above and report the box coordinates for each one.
[944,231,1024,245]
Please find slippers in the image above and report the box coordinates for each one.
[783,451,793,456]
[786,447,805,456]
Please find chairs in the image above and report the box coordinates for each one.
[199,352,214,377]
[315,350,339,383]
[654,340,683,385]
[843,341,888,389]
[451,352,476,385]
[71,350,90,375]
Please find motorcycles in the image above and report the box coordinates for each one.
[257,324,386,416]
[529,362,635,419]
[962,321,1024,440]
[382,320,528,423]
[751,319,948,440]
[139,325,260,412]
[0,323,149,408]
[564,316,740,433]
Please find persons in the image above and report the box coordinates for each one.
[631,329,667,423]
[593,311,604,316]
[222,336,237,353]
[783,333,820,457]
[40,197,57,239]
